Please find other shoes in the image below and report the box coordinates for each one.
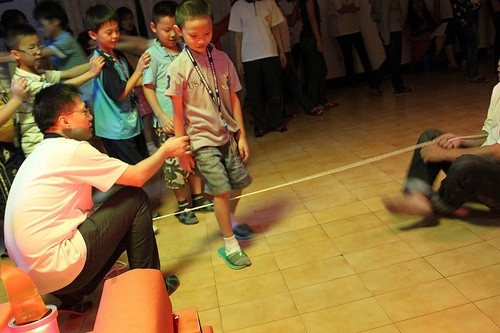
[449,63,464,72]
[394,86,415,94]
[254,129,265,138]
[323,99,337,109]
[308,107,322,115]
[469,75,491,83]
[366,89,384,96]
[276,125,287,132]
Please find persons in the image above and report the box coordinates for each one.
[379,60,500,219]
[164,0,256,269]
[142,0,217,224]
[213,0,500,137]
[5,84,192,313]
[0,0,156,259]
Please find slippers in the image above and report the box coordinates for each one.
[231,222,254,240]
[193,197,215,212]
[162,273,179,294]
[54,301,93,317]
[174,208,199,225]
[216,246,249,269]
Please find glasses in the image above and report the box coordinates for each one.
[8,44,45,56]
[59,108,91,117]
